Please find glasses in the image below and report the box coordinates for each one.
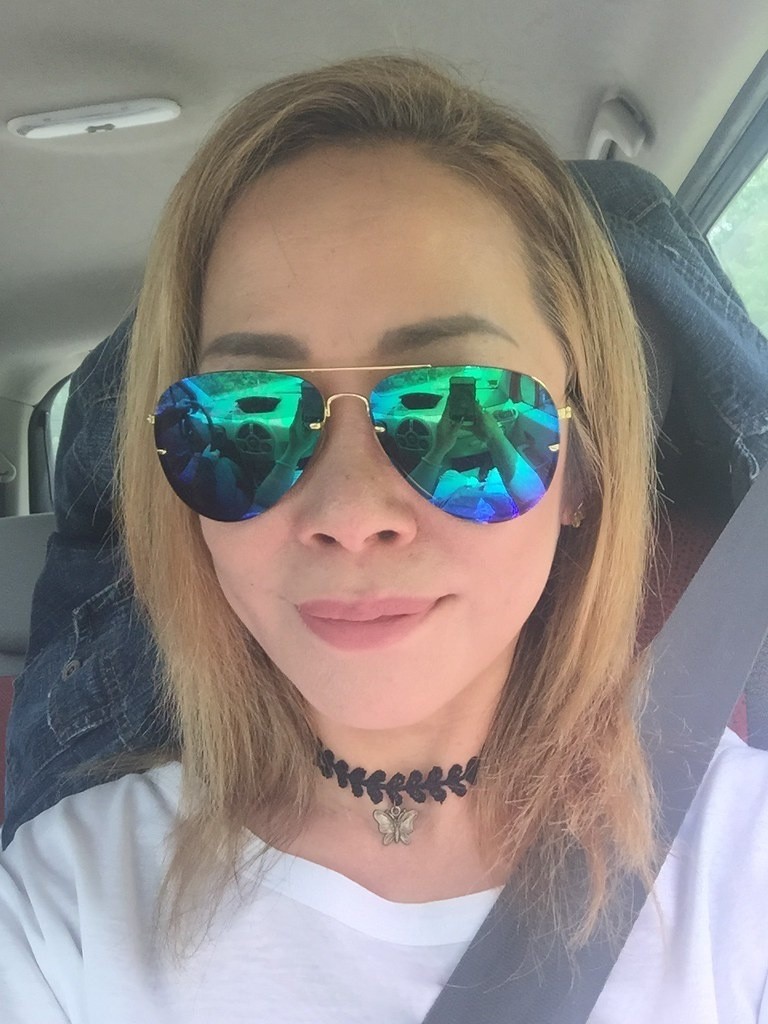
[148,364,575,523]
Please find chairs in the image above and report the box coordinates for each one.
[0,512,60,672]
[0,156,768,857]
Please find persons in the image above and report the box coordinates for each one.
[0,53,765,1024]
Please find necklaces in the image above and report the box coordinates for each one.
[296,733,483,848]
